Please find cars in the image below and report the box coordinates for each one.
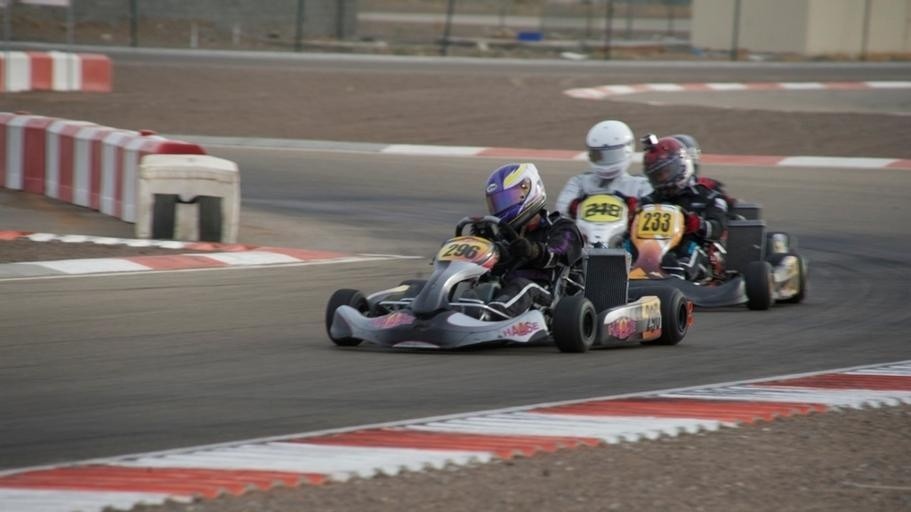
[322,194,694,354]
[566,194,808,311]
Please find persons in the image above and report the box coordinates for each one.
[672,135,735,195]
[374,163,586,320]
[556,120,653,248]
[630,136,728,282]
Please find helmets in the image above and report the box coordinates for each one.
[584,120,635,181]
[644,136,695,197]
[484,163,548,232]
[672,133,701,176]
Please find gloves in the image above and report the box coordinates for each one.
[684,213,705,238]
[508,237,545,267]
